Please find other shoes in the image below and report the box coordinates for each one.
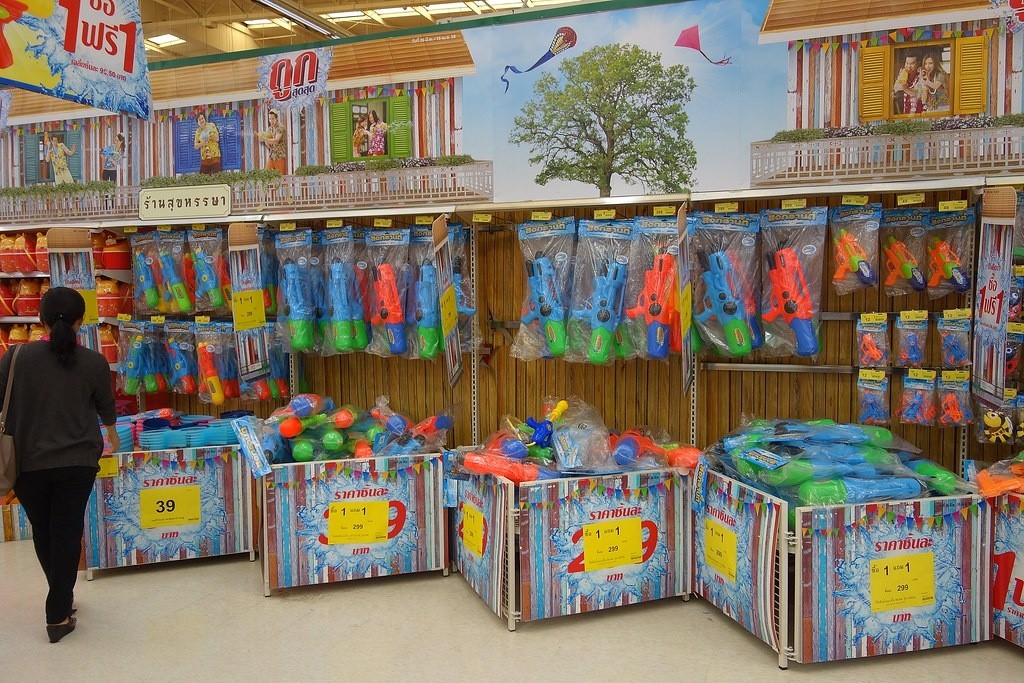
[46,609,77,643]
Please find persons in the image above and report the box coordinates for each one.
[0,286,118,641]
[258,109,287,174]
[353,110,387,157]
[194,113,221,175]
[100,133,125,181]
[897,52,950,114]
[45,135,76,185]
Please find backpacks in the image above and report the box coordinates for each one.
[0,433,16,497]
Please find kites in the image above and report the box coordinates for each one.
[500,27,577,93]
[674,26,733,66]
